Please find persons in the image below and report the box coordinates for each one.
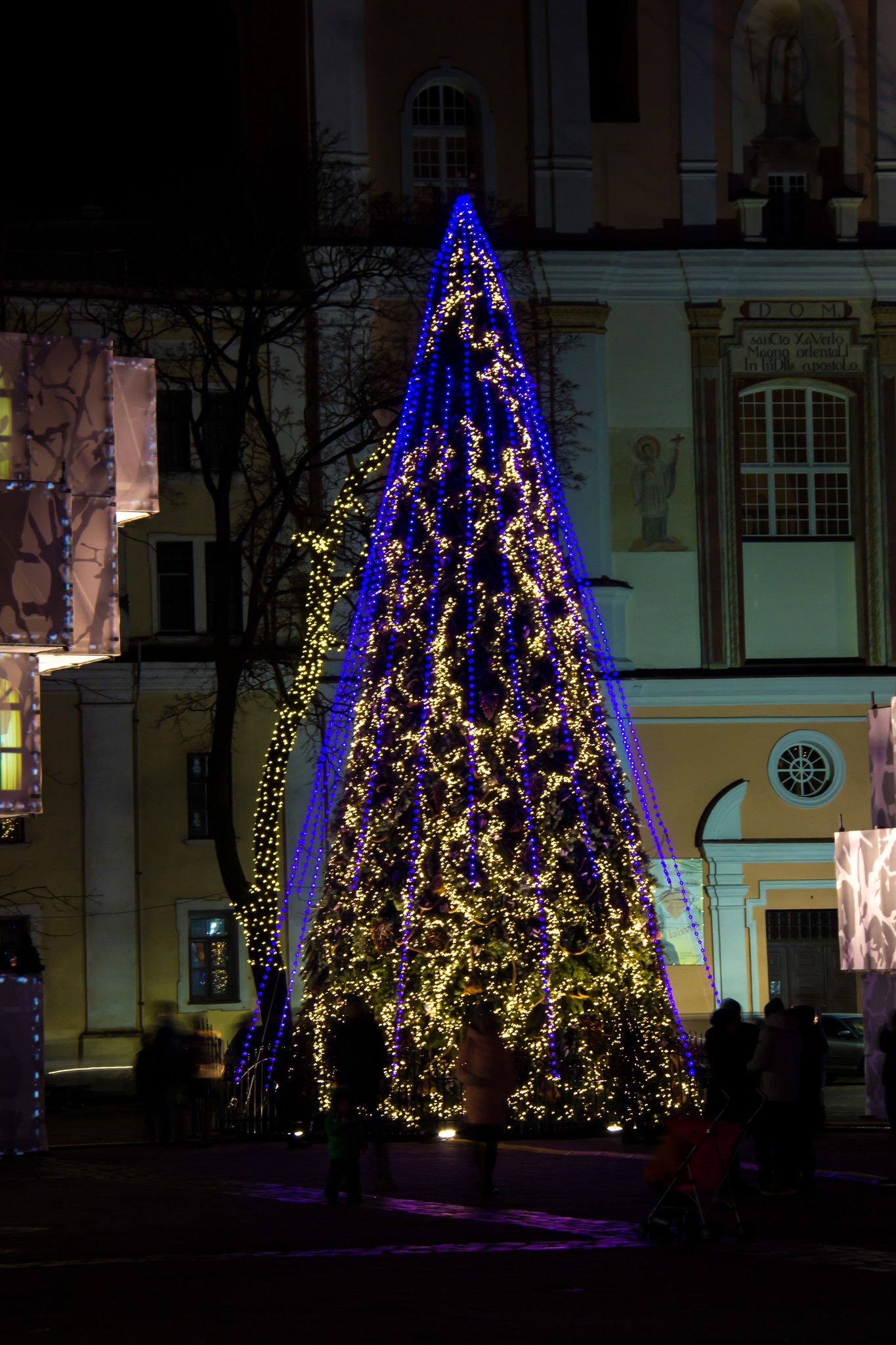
[693,989,831,1243]
[131,1002,293,1151]
[319,1082,363,1204]
[876,1007,896,1131]
[451,1011,518,1212]
[318,993,401,1206]
